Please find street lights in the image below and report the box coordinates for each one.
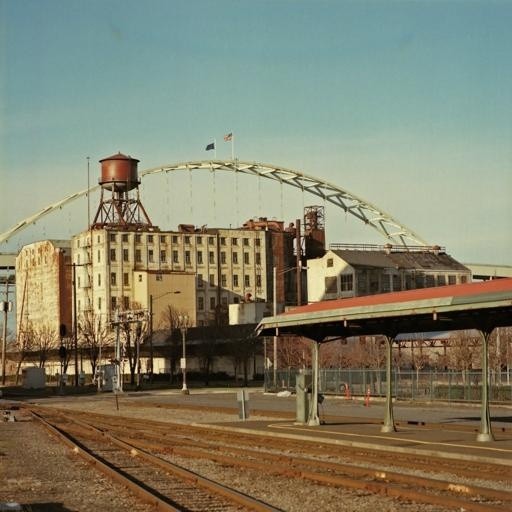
[175,315,192,396]
[0,300,14,385]
